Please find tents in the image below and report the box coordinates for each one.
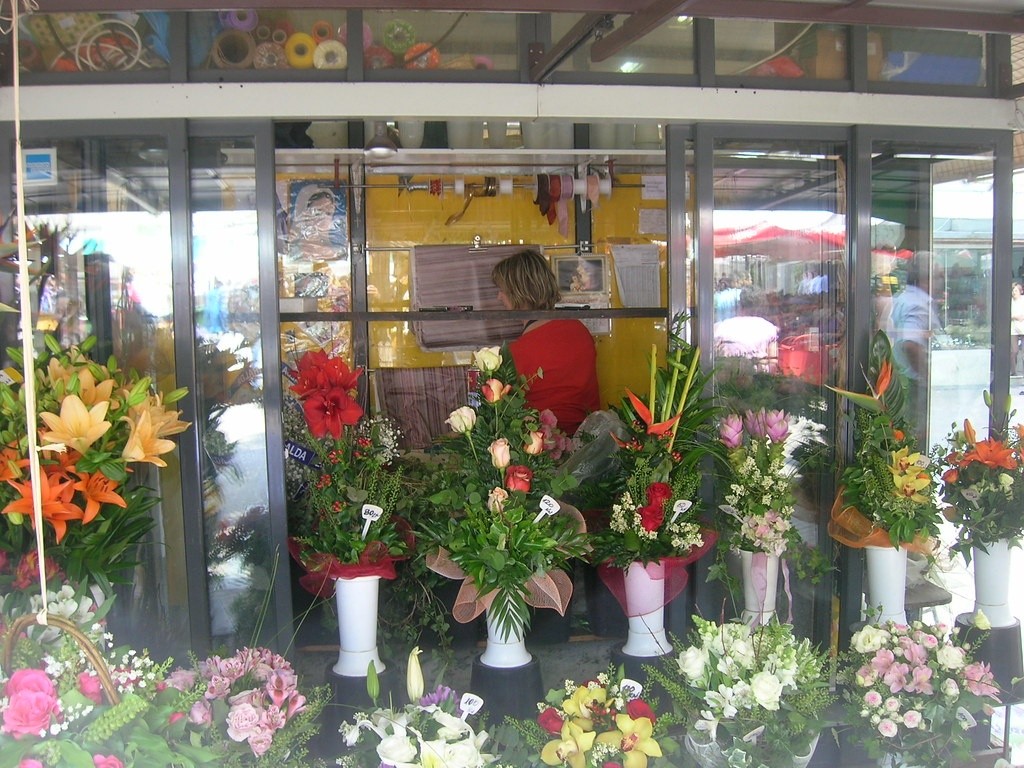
[714,211,905,259]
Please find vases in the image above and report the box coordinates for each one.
[862,544,904,633]
[325,578,396,678]
[739,546,778,628]
[622,563,672,656]
[970,525,1017,628]
[479,585,532,667]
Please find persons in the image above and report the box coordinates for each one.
[713,250,947,446]
[492,251,600,437]
[1009,282,1024,375]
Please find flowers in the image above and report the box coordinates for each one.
[0,321,1024,768]
